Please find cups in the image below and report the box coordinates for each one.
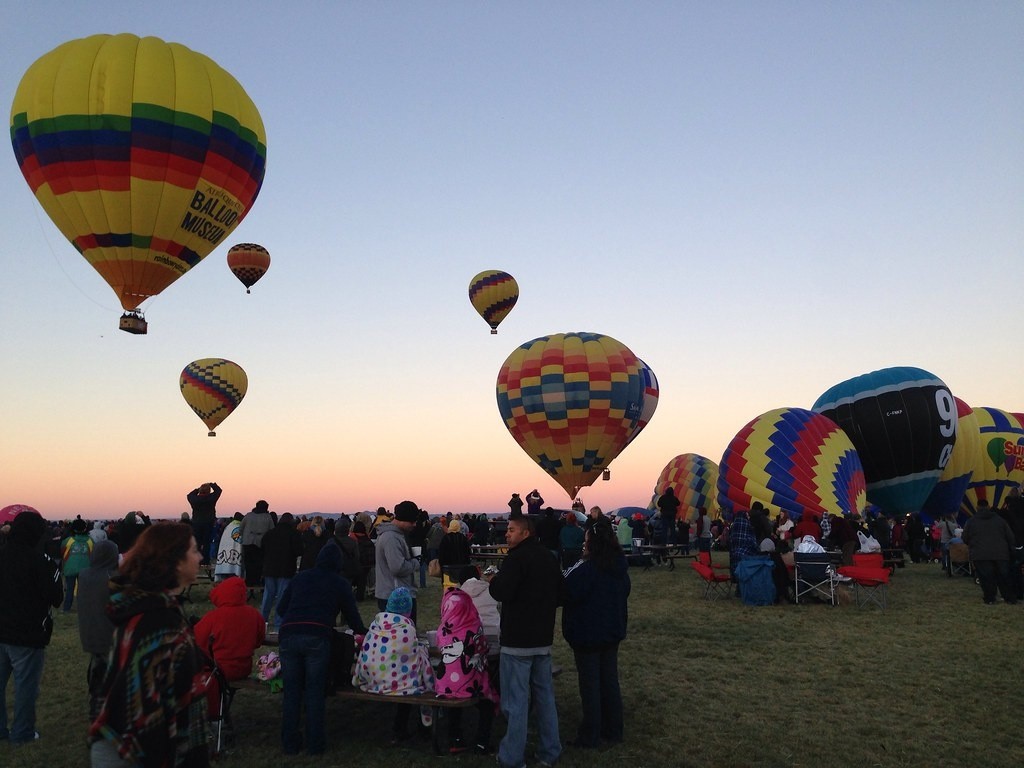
[636,540,641,547]
[426,630,437,647]
[412,547,421,564]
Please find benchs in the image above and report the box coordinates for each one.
[883,559,901,564]
[473,555,505,560]
[529,667,562,713]
[466,552,510,557]
[221,675,473,756]
[663,553,699,570]
[246,586,265,601]
[623,553,653,572]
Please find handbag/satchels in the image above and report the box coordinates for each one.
[856,530,881,554]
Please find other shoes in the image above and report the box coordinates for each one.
[33,730,41,739]
[566,736,592,750]
[533,750,553,768]
[1004,598,1018,605]
[665,562,668,567]
[495,753,527,768]
[473,743,497,756]
[599,737,624,744]
[909,560,914,564]
[983,597,996,605]
[447,740,473,753]
[390,733,409,747]
[420,732,432,742]
[658,563,662,567]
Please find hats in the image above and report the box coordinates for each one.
[385,587,413,617]
[752,501,763,510]
[394,500,419,519]
[634,513,642,520]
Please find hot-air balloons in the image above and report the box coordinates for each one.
[650,453,730,530]
[178,357,249,436]
[8,32,269,336]
[468,269,520,334]
[227,242,271,294]
[918,393,1024,533]
[494,331,646,516]
[714,407,869,539]
[811,365,958,540]
[602,356,660,482]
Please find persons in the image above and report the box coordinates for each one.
[43,506,211,768]
[183,480,377,756]
[606,486,729,567]
[353,489,632,768]
[1,505,66,742]
[866,487,1024,604]
[728,499,870,607]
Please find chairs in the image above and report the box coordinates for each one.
[735,556,778,605]
[947,543,972,576]
[692,562,731,600]
[699,549,730,573]
[853,554,886,612]
[792,550,839,607]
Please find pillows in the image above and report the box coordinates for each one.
[834,564,891,583]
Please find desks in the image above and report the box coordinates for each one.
[186,563,215,603]
[632,543,689,571]
[262,634,501,673]
[881,548,905,576]
[471,544,510,571]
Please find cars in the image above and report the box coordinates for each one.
[538,508,589,528]
